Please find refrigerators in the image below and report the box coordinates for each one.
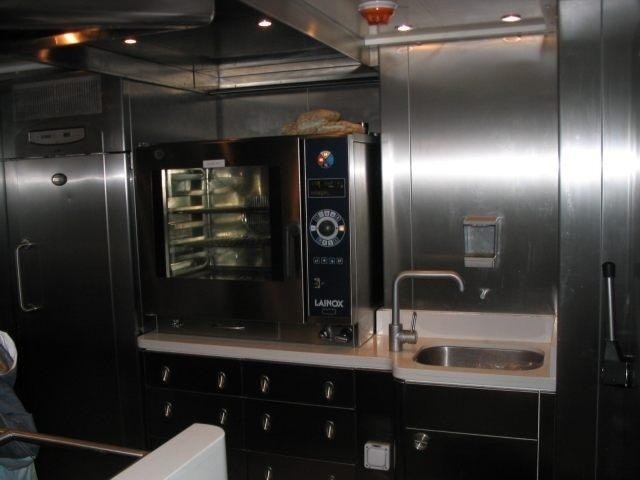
[0,69,219,480]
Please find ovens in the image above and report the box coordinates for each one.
[139,132,380,350]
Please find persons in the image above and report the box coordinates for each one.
[0,330,41,480]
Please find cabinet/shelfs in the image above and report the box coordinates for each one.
[391,378,557,480]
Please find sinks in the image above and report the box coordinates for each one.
[413,342,546,371]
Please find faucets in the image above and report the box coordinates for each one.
[391,269,466,325]
